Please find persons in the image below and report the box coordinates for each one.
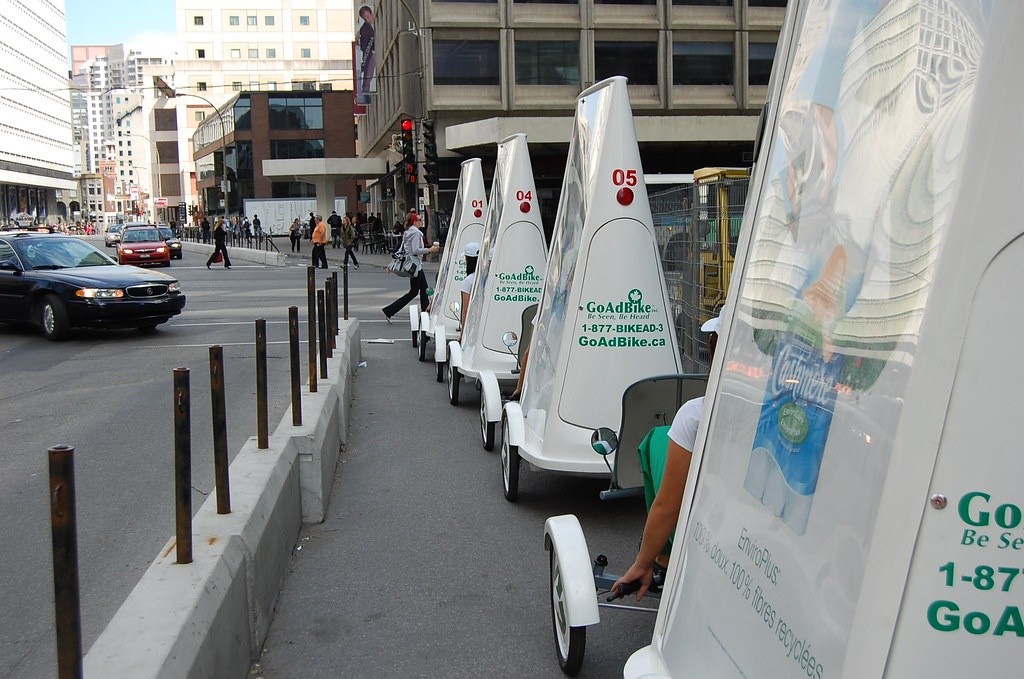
[340,217,360,270]
[312,214,328,269]
[460,241,480,340]
[169,207,416,253]
[359,6,375,92]
[56,221,94,237]
[610,305,725,601]
[381,213,440,324]
[206,221,231,269]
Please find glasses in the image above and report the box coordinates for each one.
[416,219,422,221]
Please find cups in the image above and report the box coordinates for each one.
[433,241,439,250]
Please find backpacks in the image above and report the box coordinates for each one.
[244,219,249,229]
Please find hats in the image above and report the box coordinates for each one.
[460,242,480,256]
[701,305,725,335]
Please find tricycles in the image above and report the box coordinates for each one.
[407,0,1023,678]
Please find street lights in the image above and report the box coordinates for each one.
[176,93,229,219]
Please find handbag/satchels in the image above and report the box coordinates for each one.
[348,225,359,242]
[213,253,223,263]
[387,242,419,277]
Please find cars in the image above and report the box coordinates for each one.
[115,225,171,267]
[0,225,186,343]
[158,226,182,259]
[105,225,122,246]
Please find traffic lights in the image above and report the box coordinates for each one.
[405,165,417,185]
[422,118,440,186]
[400,119,417,163]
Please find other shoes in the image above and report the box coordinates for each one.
[312,266,318,268]
[354,264,360,270]
[380,309,393,324]
[340,265,345,270]
[319,266,327,269]
[639,532,667,586]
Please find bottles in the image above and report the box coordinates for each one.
[426,287,434,295]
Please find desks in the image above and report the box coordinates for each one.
[384,233,403,249]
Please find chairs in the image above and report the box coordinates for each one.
[361,234,392,256]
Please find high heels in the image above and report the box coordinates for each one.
[205,264,211,269]
[224,264,231,269]
